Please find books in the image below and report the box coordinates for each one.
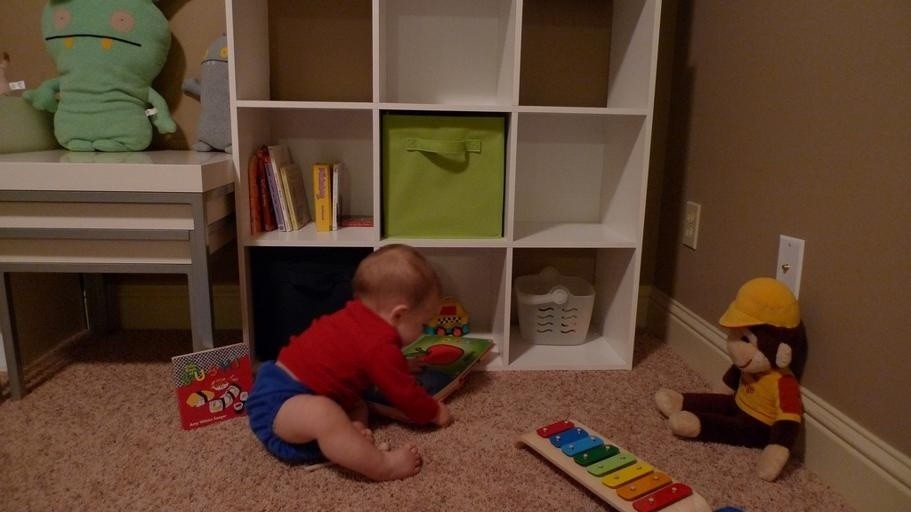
[173,343,253,431]
[372,332,495,416]
[248,145,342,236]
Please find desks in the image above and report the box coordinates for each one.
[1,150,234,401]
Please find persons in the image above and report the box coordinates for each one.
[248,244,453,480]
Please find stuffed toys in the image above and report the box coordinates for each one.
[21,1,177,152]
[182,34,233,153]
[654,277,805,482]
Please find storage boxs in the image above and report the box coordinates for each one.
[381,113,507,240]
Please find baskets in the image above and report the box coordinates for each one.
[515,266,595,345]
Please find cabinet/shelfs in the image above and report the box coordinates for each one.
[224,0,661,372]
